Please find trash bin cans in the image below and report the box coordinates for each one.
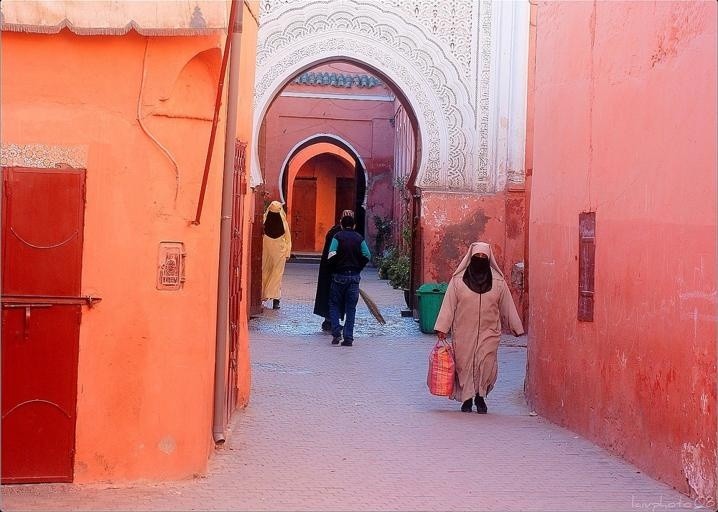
[414,283,448,334]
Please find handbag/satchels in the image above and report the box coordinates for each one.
[427,336,455,395]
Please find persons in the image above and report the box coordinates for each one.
[327,216,372,347]
[313,208,354,329]
[434,242,525,408]
[261,201,292,310]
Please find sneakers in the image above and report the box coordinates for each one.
[322,321,353,346]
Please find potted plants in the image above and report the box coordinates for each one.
[399,257,411,308]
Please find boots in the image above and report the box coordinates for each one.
[273,300,280,309]
[462,392,487,414]
